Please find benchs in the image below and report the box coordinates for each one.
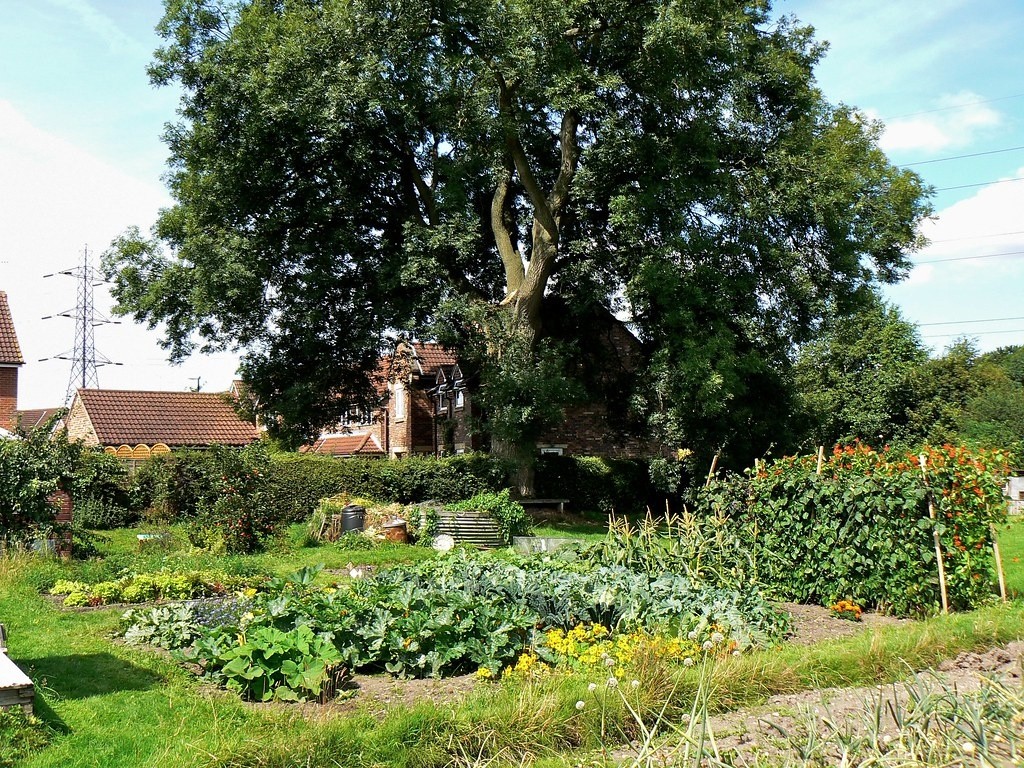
[516,497,570,514]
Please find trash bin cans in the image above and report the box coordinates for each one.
[341,505,367,537]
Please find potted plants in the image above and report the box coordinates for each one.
[407,486,536,549]
